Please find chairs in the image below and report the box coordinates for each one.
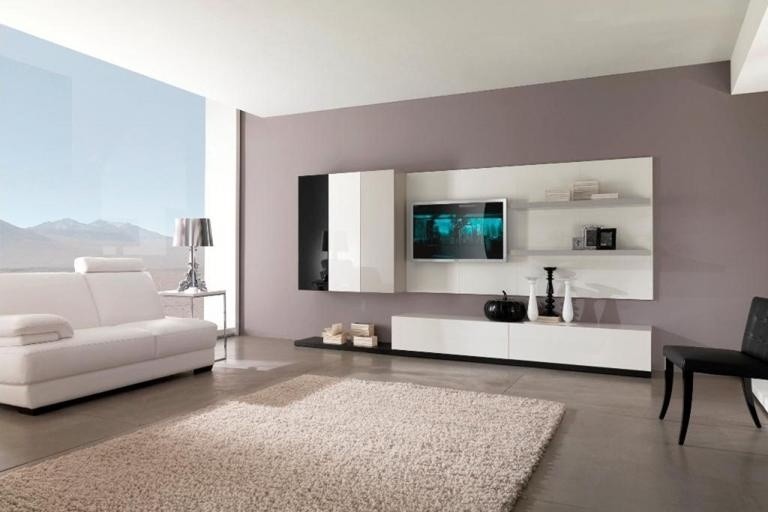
[659,295,768,445]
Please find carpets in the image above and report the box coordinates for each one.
[1,373,565,512]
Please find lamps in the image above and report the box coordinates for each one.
[173,218,213,292]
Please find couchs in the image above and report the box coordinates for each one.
[0,257,218,414]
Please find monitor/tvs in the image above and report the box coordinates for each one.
[409,198,508,263]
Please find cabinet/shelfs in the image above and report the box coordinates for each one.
[327,168,407,294]
[405,156,654,300]
[292,312,652,379]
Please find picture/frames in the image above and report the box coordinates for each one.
[572,237,584,250]
[596,228,616,250]
[583,226,597,249]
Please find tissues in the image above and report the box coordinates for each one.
[353,335,377,347]
[321,323,348,344]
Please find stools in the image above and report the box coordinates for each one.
[156,289,227,362]
